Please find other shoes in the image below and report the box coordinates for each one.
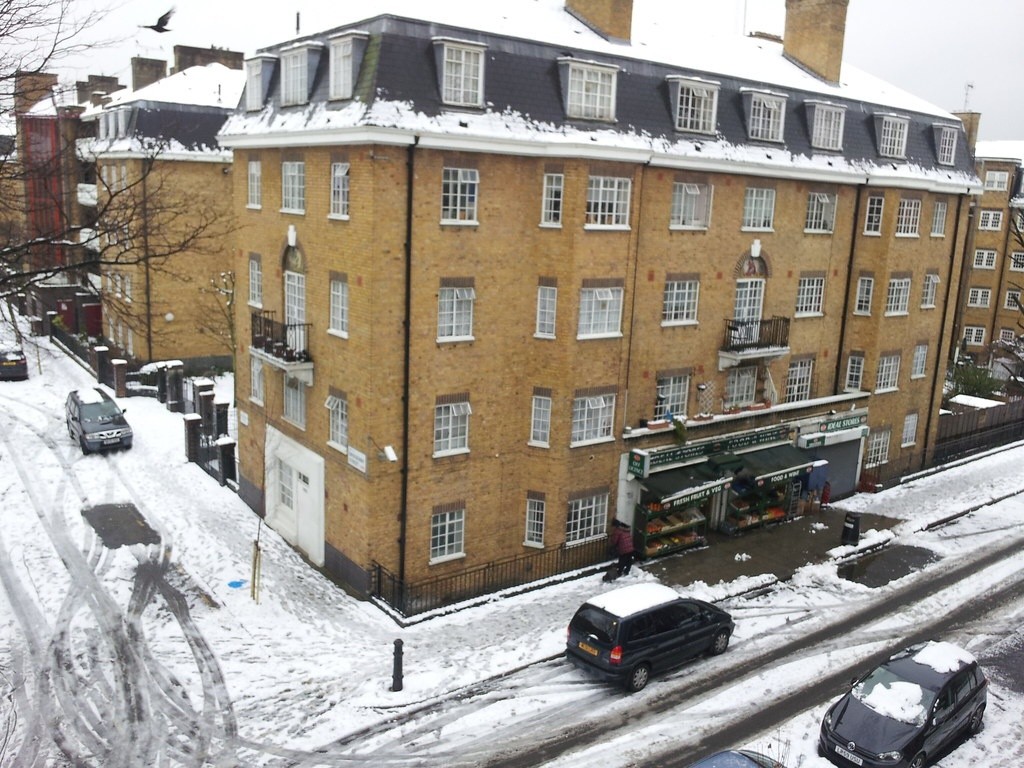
[623,569,628,575]
[617,570,623,575]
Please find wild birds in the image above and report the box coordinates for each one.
[138,6,175,33]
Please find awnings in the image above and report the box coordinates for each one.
[635,462,733,510]
[740,444,814,488]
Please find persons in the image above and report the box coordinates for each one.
[820,481,830,506]
[609,517,632,574]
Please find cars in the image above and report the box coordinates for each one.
[0,349,29,382]
[566,583,736,692]
[818,638,988,768]
[684,748,786,768]
[66,388,133,455]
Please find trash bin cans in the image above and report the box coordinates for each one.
[839,511,861,547]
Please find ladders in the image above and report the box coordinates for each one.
[784,480,802,524]
